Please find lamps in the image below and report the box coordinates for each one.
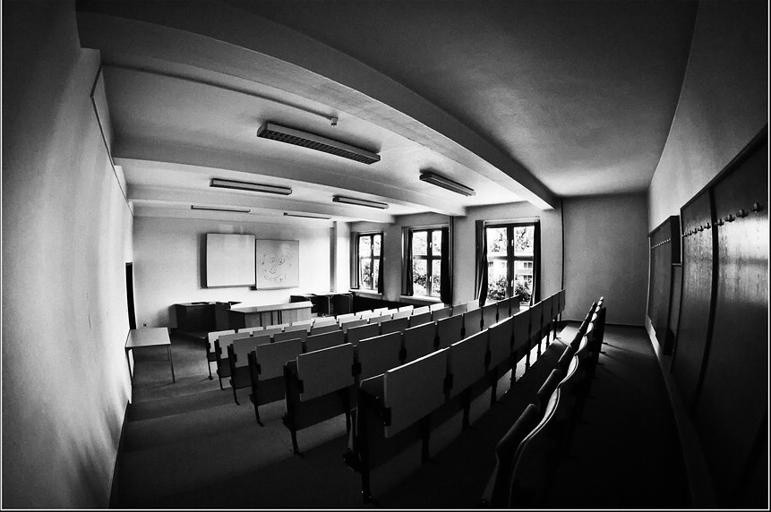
[209,178,292,196]
[255,122,381,165]
[419,173,476,196]
[283,212,331,220]
[331,195,389,210]
[190,205,251,214]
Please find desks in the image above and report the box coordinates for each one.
[124,326,175,389]
[174,302,215,329]
